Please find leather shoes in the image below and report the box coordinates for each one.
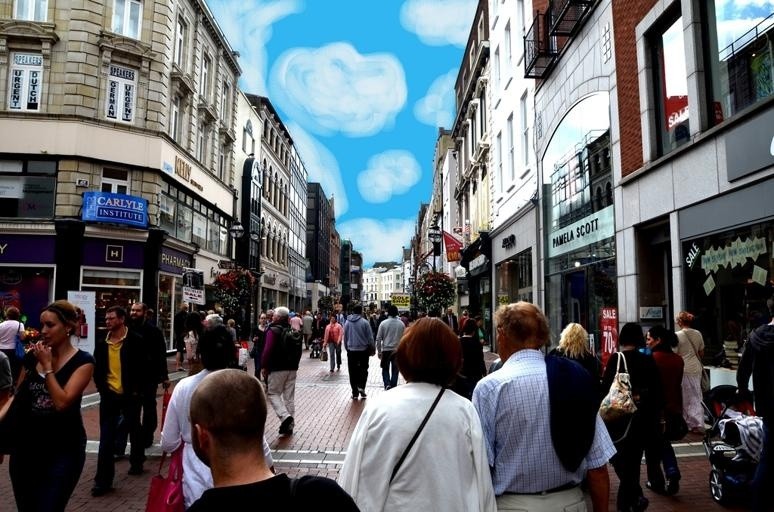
[644,480,665,495]
[665,473,680,495]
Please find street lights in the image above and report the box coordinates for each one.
[250,219,267,339]
[425,221,444,272]
[229,218,245,272]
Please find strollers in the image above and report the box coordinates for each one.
[700,382,767,502]
[309,331,324,359]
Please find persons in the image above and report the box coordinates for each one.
[737,293,774,512]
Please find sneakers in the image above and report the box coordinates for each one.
[126,461,147,475]
[386,385,391,390]
[351,394,358,400]
[358,387,367,399]
[277,415,294,435]
[91,482,113,497]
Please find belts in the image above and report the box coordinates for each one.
[497,482,580,497]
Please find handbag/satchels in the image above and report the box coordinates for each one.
[599,351,638,423]
[144,437,187,512]
[13,321,26,359]
[320,346,328,362]
[161,388,174,434]
[699,375,710,392]
